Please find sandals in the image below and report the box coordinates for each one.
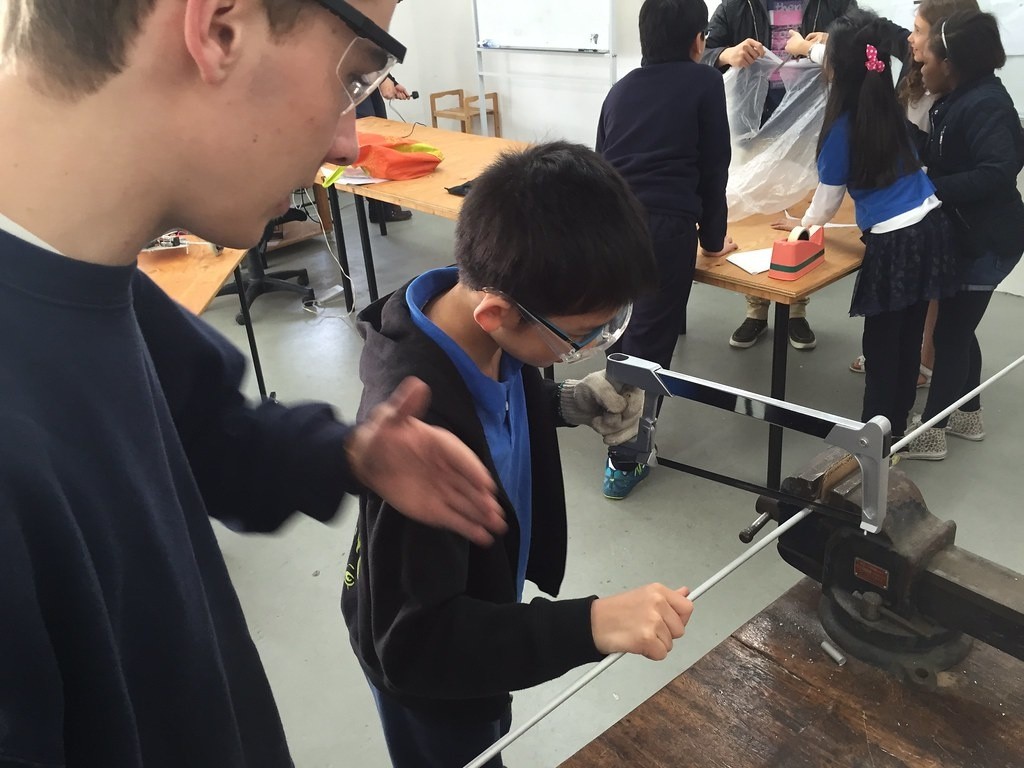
[850,354,866,373]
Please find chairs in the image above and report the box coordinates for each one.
[215,207,315,326]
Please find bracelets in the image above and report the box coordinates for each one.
[808,42,821,64]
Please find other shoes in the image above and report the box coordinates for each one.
[369,204,412,224]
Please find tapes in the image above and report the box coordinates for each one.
[787,225,812,242]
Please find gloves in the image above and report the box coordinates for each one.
[560,368,644,445]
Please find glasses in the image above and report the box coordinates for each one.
[483,286,633,365]
[326,0,407,115]
[530,311,609,354]
[702,28,711,39]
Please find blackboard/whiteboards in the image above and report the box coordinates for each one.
[472,0,616,57]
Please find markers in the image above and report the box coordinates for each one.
[576,48,598,53]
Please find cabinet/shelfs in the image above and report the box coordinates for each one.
[430,88,501,138]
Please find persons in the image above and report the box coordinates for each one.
[898,9,1023,459]
[700,0,858,348]
[352,70,412,222]
[850,0,981,390]
[0,0,504,768]
[771,11,941,470]
[595,0,740,498]
[340,141,694,767]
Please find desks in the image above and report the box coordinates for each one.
[557,576,1024,768]
[137,234,275,404]
[315,115,867,491]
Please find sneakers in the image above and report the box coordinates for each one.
[899,421,948,460]
[730,317,768,347]
[950,407,985,441]
[789,317,816,349]
[604,457,651,499]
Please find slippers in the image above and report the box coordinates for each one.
[916,365,933,387]
